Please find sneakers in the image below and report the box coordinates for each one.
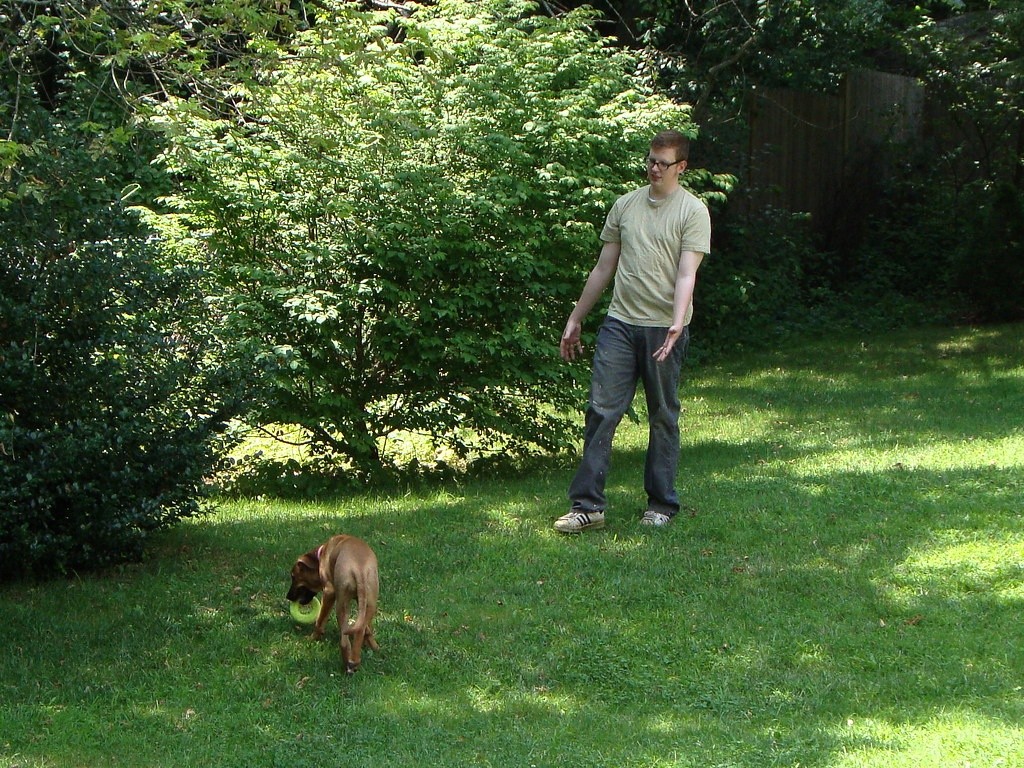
[639,509,670,526]
[553,510,605,532]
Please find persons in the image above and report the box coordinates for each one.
[552,130,710,531]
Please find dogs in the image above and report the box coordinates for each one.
[287,534,381,676]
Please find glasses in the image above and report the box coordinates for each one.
[644,157,687,170]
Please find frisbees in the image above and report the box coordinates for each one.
[289,595,322,626]
